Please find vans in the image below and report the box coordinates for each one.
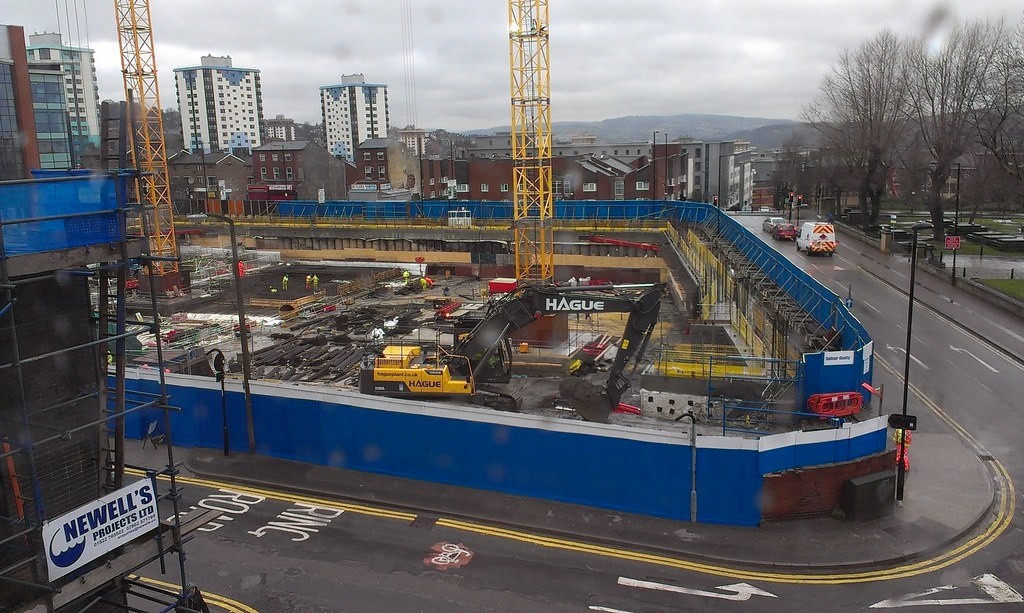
[795,221,835,256]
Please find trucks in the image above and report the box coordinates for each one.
[488,277,518,305]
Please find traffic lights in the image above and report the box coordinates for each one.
[789,192,793,202]
[745,201,747,205]
[713,196,718,206]
[797,195,802,205]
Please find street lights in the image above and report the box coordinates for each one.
[653,131,659,199]
[717,155,726,237]
[185,211,258,456]
[896,220,936,507]
[930,160,962,287]
[665,133,668,200]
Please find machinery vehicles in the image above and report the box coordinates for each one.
[354,281,670,426]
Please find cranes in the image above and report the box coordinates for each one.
[114,0,195,297]
[497,1,571,348]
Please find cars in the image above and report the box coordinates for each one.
[762,218,786,233]
[772,224,796,242]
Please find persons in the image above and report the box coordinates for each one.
[827,214,834,222]
[310,274,319,290]
[403,270,409,284]
[419,278,427,291]
[282,275,289,290]
[108,350,112,366]
[306,274,312,289]
[893,429,912,472]
[424,276,432,288]
